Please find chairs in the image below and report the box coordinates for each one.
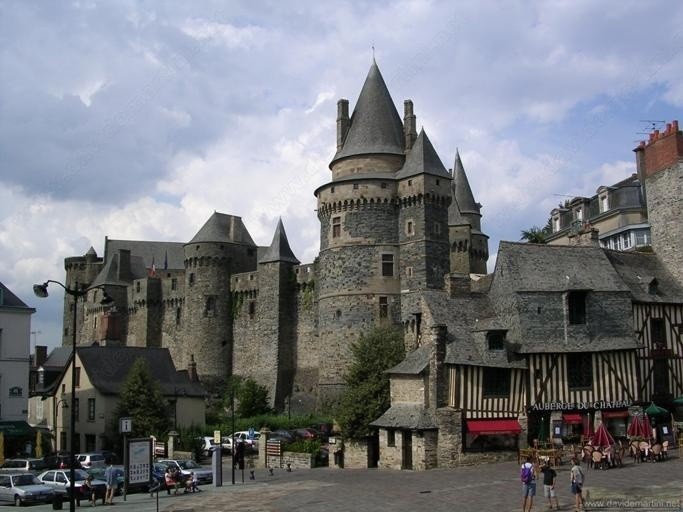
[629,436,669,463]
[581,440,625,470]
[518,438,562,470]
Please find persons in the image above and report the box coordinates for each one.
[81,457,202,507]
[569,457,587,511]
[520,456,536,511]
[538,457,560,509]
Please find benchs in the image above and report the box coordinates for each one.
[164,477,198,495]
[65,483,107,507]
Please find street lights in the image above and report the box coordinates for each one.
[30,278,115,512]
[36,388,70,456]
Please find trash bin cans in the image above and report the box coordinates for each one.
[52,493,63,510]
[334,451,343,465]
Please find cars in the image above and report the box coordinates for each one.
[0,425,318,507]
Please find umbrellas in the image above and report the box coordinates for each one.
[589,422,614,451]
[641,412,653,446]
[628,412,643,441]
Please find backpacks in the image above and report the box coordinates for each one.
[519,463,533,484]
